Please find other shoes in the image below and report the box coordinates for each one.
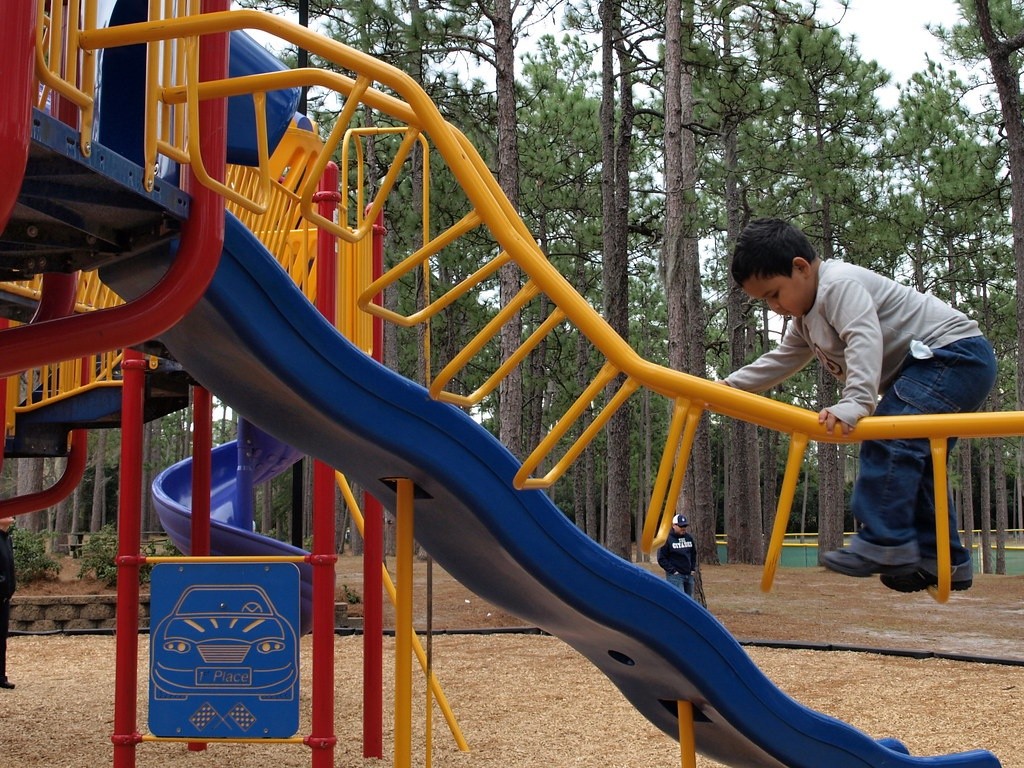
[0,676,16,689]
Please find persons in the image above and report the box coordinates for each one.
[657,514,697,602]
[0,515,17,689]
[705,216,998,593]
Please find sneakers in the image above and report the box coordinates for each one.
[880,548,974,594]
[819,538,919,578]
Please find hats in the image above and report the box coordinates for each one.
[672,513,690,527]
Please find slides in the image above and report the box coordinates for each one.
[150,399,318,638]
[101,204,1010,768]
